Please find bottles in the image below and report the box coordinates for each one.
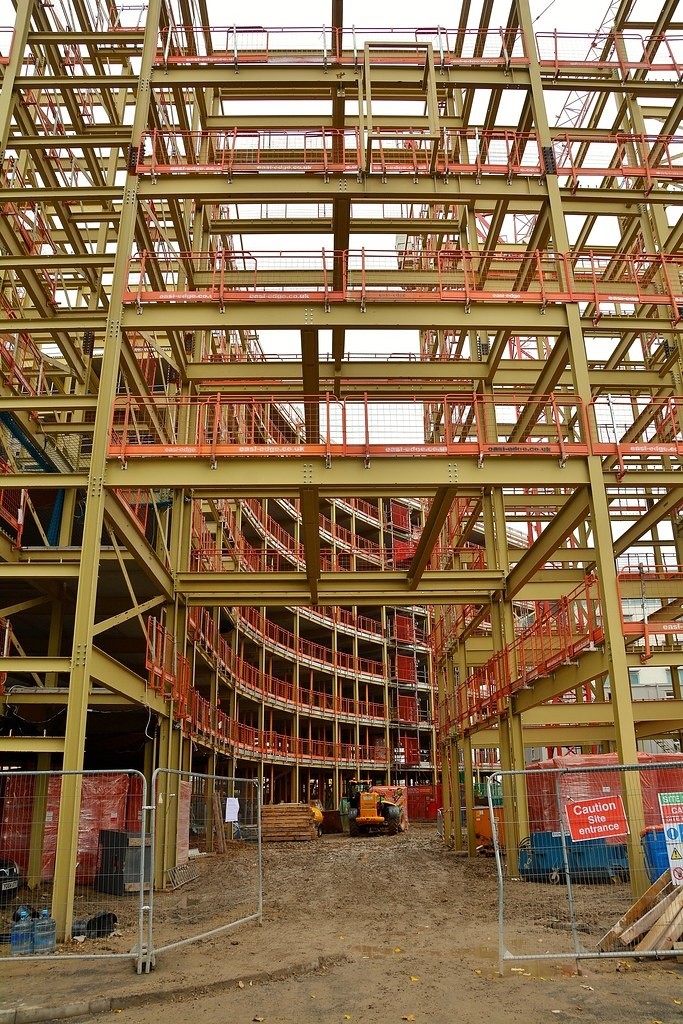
[10,911,34,954]
[33,910,57,953]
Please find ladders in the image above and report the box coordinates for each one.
[167,860,201,890]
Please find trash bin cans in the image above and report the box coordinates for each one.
[640,823,683,885]
[340,797,349,815]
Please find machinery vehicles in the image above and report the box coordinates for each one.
[346,777,403,838]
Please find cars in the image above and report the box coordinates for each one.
[0,854,20,910]
